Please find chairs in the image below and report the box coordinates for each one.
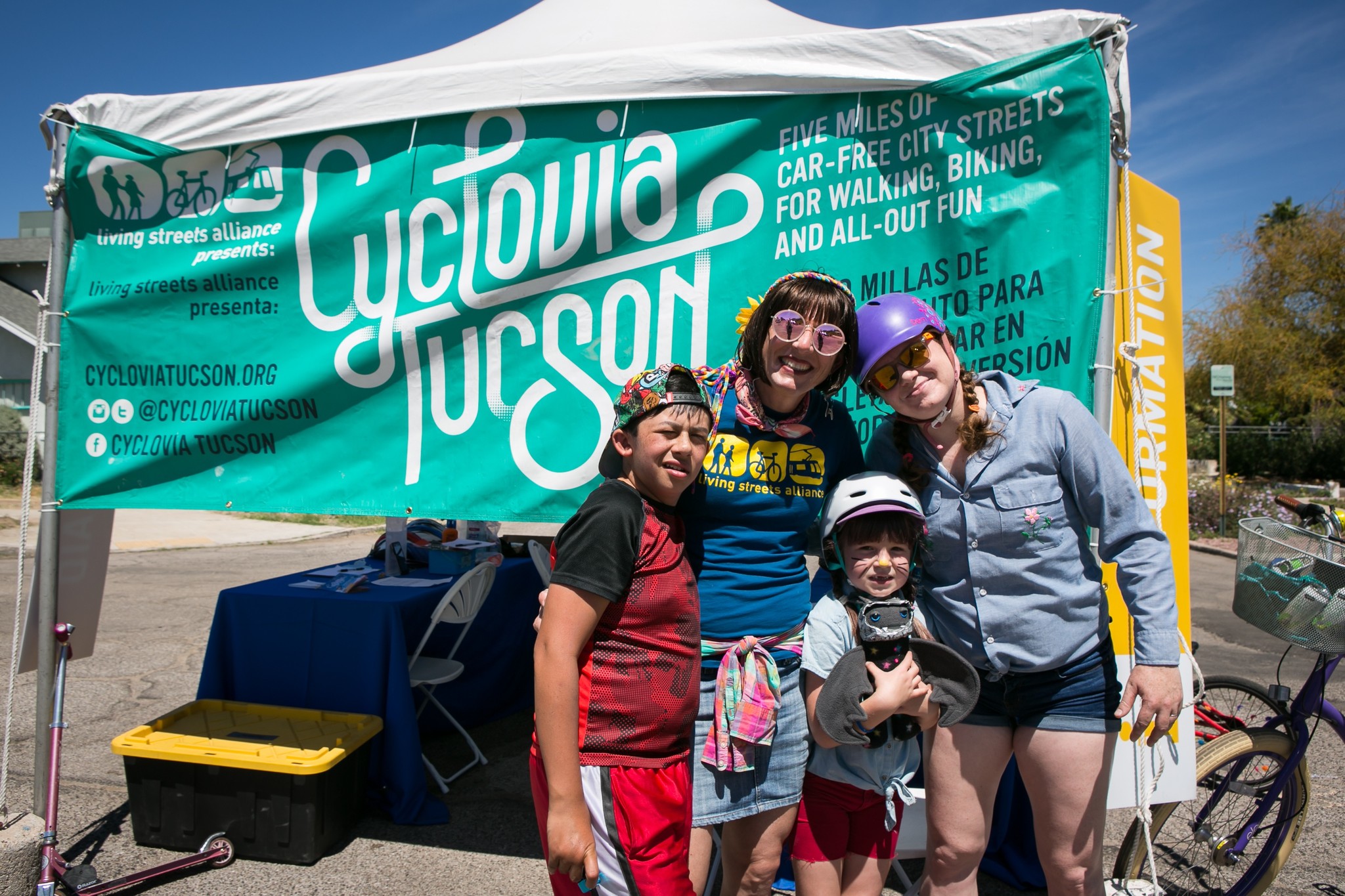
[408,561,496,789]
[528,540,552,588]
[704,823,796,896]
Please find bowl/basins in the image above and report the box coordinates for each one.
[511,543,524,554]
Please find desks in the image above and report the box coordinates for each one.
[196,559,546,828]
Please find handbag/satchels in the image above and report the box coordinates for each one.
[466,519,502,552]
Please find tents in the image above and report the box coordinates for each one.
[33,0,1132,819]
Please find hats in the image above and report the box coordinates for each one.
[599,364,716,479]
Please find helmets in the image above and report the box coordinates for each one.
[850,292,947,395]
[821,471,927,571]
[407,519,447,543]
[370,528,429,561]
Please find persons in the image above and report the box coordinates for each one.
[673,272,869,896]
[786,472,944,896]
[524,365,717,896]
[848,290,1184,896]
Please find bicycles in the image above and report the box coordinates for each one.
[1184,640,1292,794]
[1092,495,1345,896]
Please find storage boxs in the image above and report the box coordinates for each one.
[111,702,384,866]
[423,544,486,574]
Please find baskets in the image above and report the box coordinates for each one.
[1232,516,1345,654]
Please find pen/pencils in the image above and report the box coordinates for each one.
[456,542,481,546]
[337,568,364,572]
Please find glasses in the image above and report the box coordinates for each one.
[867,332,936,390]
[770,310,848,356]
